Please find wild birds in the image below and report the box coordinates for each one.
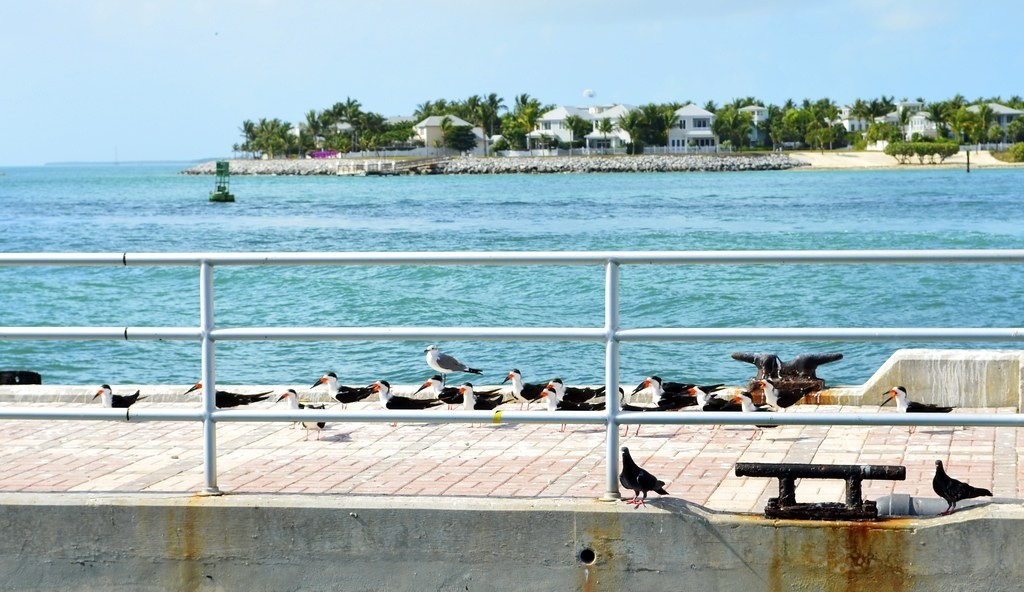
[301,404,327,441]
[93,384,139,407]
[530,378,606,432]
[367,380,443,427]
[414,374,503,410]
[932,459,993,516]
[502,368,548,410]
[452,382,514,427]
[424,345,482,385]
[184,379,274,409]
[311,372,376,409]
[276,389,311,429]
[880,385,957,434]
[631,375,726,411]
[748,379,818,412]
[619,387,668,436]
[719,391,779,440]
[618,446,668,504]
[677,386,774,431]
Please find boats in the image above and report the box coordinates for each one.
[208,160,235,202]
